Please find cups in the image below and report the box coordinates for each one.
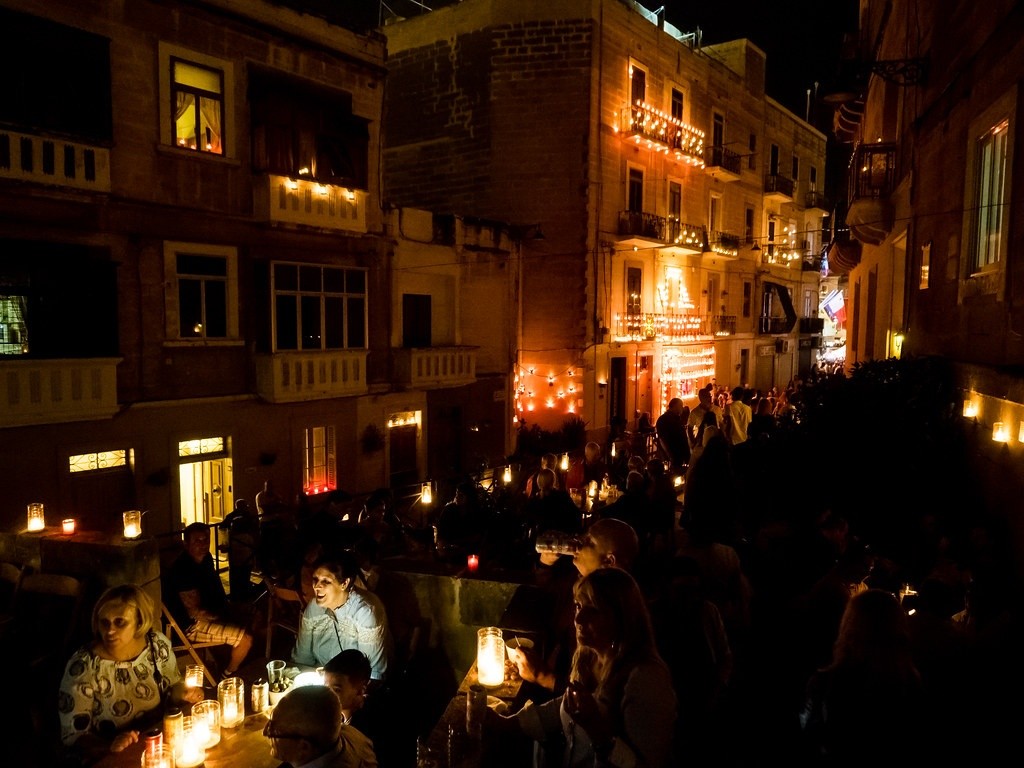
[466,553,479,579]
[295,671,325,688]
[191,699,222,749]
[416,626,507,768]
[184,664,205,687]
[141,716,207,768]
[265,660,286,683]
[532,530,586,565]
[569,476,621,513]
[270,680,294,706]
[26,502,142,540]
[844,581,918,615]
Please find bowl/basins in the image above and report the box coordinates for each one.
[505,637,534,662]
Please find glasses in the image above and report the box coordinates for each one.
[231,537,255,550]
[263,719,316,741]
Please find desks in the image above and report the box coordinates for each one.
[370,565,530,690]
[427,585,560,768]
[93,657,384,768]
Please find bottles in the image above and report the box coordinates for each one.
[145,727,163,761]
[251,677,269,713]
[162,706,183,744]
[217,677,246,729]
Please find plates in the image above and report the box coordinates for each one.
[266,706,276,719]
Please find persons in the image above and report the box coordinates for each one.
[533,515,641,694]
[220,478,394,768]
[479,567,680,768]
[404,360,1001,749]
[168,522,255,681]
[58,584,205,768]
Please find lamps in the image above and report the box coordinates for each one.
[751,240,761,251]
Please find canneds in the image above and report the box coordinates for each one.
[143,728,163,766]
[251,678,269,712]
[466,685,487,724]
[162,707,184,744]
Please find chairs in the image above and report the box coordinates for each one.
[31,439,733,768]
